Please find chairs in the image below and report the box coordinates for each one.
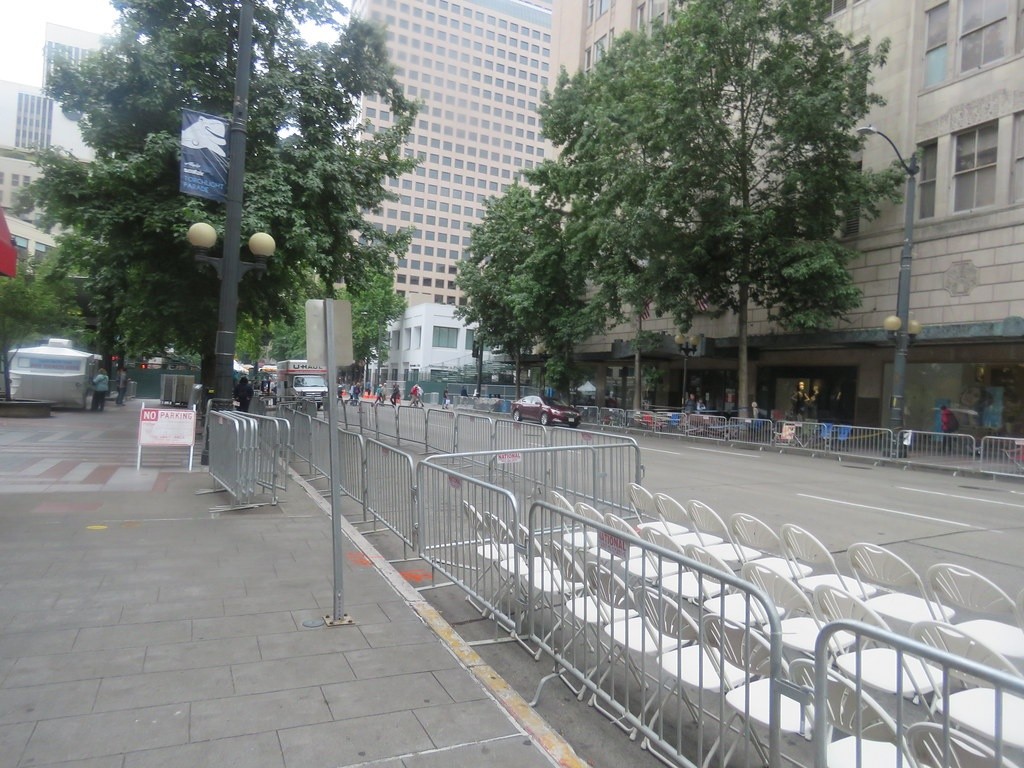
[831,427,852,452]
[462,480,1024,768]
[670,414,679,429]
[818,422,833,445]
[1001,440,1024,474]
[794,419,817,448]
[773,423,796,446]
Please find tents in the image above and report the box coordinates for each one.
[579,381,597,396]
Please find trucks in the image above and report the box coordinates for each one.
[276,359,329,408]
[239,362,278,396]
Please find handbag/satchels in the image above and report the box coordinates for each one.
[445,399,450,405]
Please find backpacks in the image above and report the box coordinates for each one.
[948,413,959,429]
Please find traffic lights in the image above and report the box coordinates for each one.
[141,364,146,369]
[111,356,115,361]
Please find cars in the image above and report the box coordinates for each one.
[511,395,582,428]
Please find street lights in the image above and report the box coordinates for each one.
[188,223,277,467]
[858,127,921,457]
[676,334,699,415]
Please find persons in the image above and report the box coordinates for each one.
[115,367,129,407]
[409,384,425,408]
[473,387,479,396]
[810,385,822,417]
[955,387,992,427]
[1006,416,1017,433]
[376,383,388,404]
[89,368,111,412]
[695,398,706,414]
[685,393,696,411]
[338,384,345,405]
[272,382,278,405]
[390,382,398,405]
[235,377,254,412]
[442,386,449,409]
[940,406,952,452]
[345,383,361,406]
[460,386,468,396]
[792,381,811,423]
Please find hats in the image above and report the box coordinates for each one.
[379,384,382,386]
